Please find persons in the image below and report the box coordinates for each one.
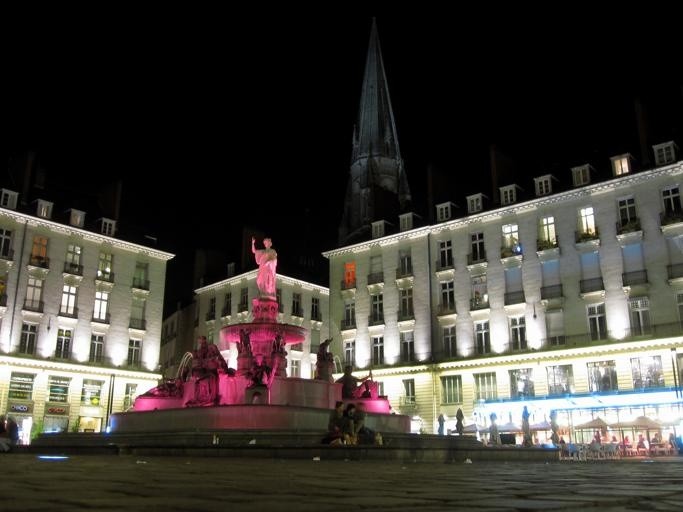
[0,415,6,433]
[252,236,278,300]
[328,400,383,446]
[560,430,675,450]
[0,415,19,453]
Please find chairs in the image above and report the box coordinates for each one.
[557,441,671,461]
